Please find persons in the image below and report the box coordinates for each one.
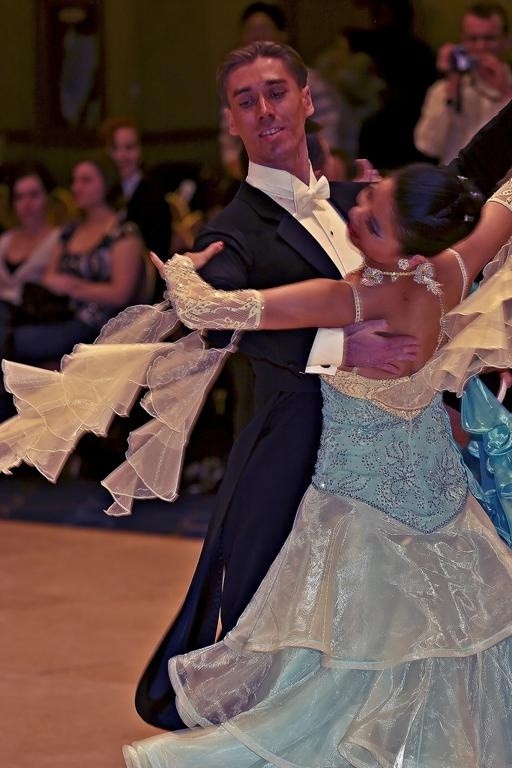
[131,31,512,730]
[146,163,510,768]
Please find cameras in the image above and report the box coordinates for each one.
[448,44,475,72]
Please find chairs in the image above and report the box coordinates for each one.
[48,183,222,310]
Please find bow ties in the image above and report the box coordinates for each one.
[293,174,331,214]
